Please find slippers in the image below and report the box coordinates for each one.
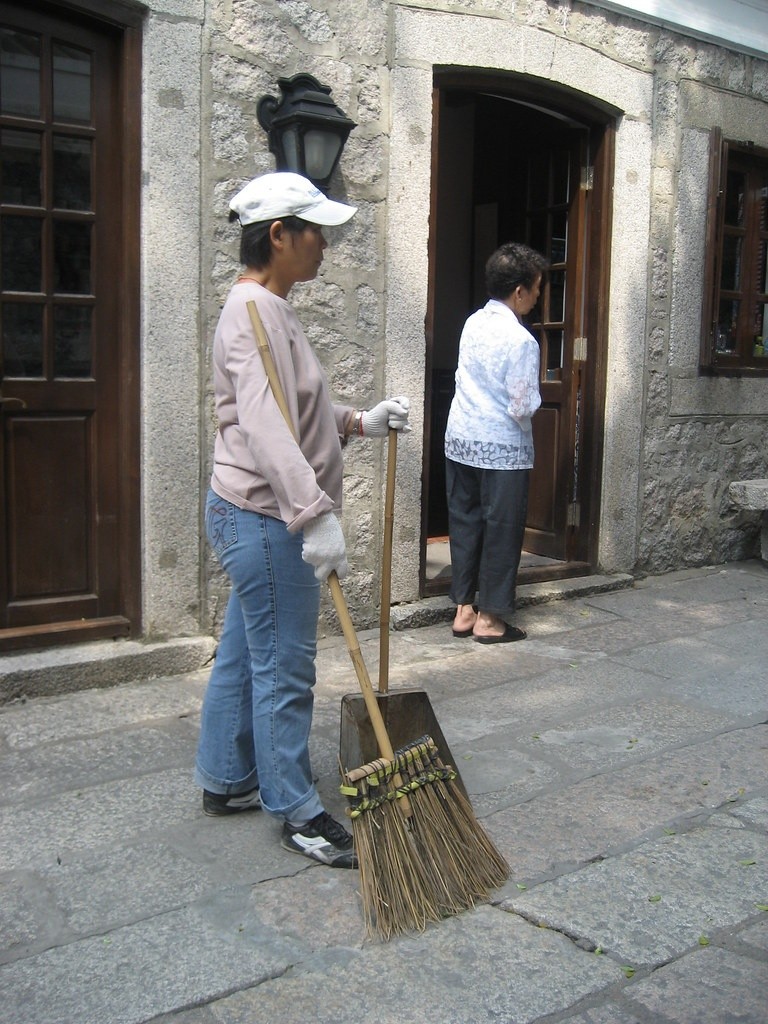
[453,608,479,638]
[478,621,526,644]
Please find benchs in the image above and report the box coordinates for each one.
[729,478,768,564]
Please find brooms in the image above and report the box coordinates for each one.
[246,301,516,945]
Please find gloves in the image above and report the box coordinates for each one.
[301,511,347,580]
[360,396,412,438]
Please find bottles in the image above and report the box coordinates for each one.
[753,336,764,356]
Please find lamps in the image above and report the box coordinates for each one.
[256,72,359,198]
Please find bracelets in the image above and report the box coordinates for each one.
[352,412,362,435]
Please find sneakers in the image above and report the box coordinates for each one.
[280,811,359,870]
[202,785,261,817]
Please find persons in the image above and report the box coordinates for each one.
[444,242,549,638]
[193,172,412,870]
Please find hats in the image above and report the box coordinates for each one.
[229,172,359,227]
